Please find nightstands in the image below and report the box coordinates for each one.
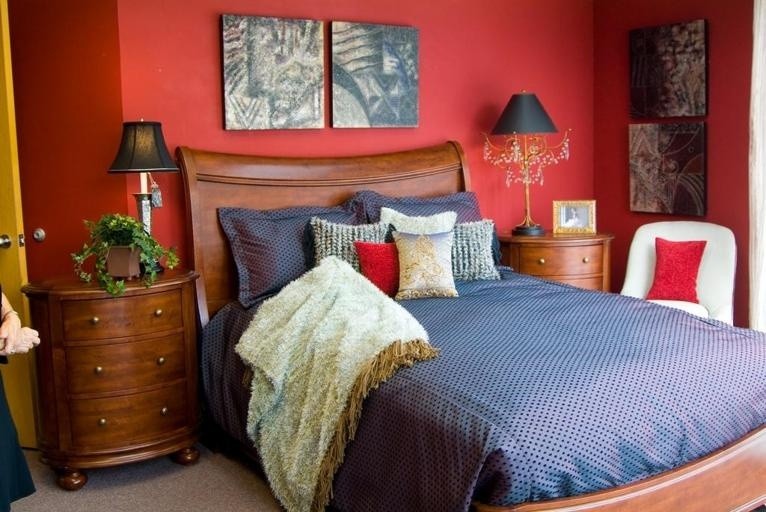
[494,225,612,293]
[23,271,202,488]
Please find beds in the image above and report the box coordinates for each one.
[172,138,766,512]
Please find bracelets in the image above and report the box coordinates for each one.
[2,310,20,318]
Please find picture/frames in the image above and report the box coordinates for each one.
[554,201,597,235]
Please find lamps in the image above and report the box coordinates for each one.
[484,92,572,236]
[108,122,180,271]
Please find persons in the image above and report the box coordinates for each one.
[566,208,581,226]
[0,285,43,510]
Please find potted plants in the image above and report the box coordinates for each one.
[74,215,180,295]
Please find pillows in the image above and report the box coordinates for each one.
[646,237,707,303]
[215,189,500,305]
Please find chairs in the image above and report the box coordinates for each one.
[618,221,736,328]
